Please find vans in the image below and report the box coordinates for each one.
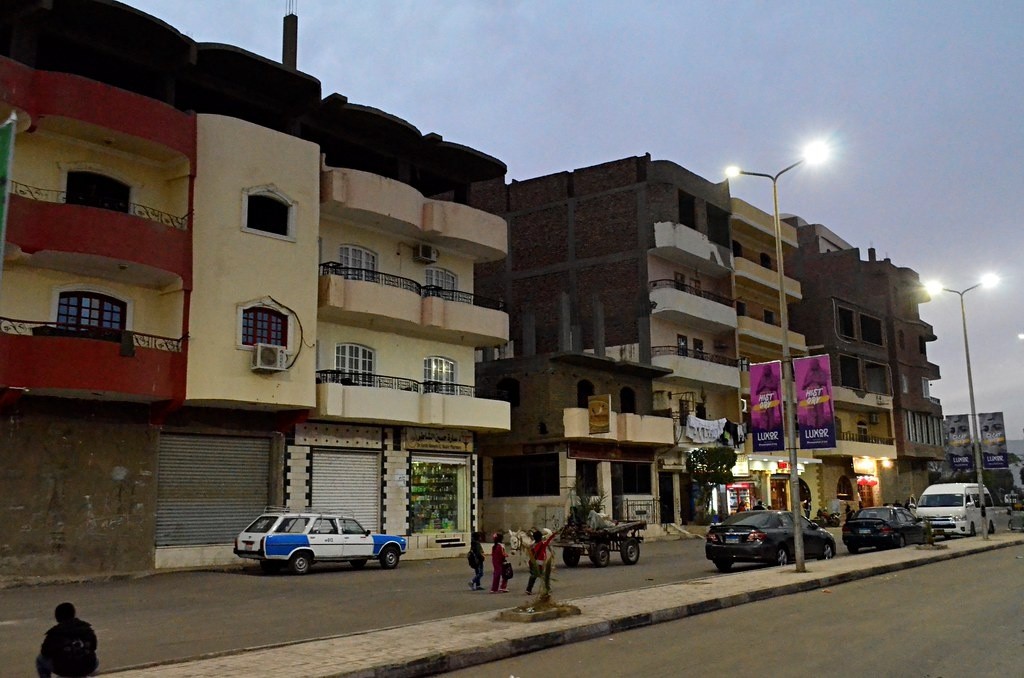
[916,483,997,536]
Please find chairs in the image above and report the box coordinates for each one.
[868,511,877,518]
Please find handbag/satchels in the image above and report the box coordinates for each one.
[500,557,513,581]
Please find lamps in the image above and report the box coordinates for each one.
[849,462,854,474]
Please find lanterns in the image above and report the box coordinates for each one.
[857,476,878,487]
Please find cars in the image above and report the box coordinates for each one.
[705,509,836,568]
[232,506,407,575]
[842,507,933,554]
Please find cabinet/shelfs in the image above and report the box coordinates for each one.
[412,472,457,533]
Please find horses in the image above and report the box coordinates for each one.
[509,528,553,574]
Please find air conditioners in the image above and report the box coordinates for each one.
[415,242,436,262]
[870,414,881,422]
[252,342,288,373]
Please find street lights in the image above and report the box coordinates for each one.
[922,270,1004,543]
[725,143,830,574]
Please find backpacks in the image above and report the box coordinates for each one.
[468,543,481,569]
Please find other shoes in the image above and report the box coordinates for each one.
[525,591,533,595]
[468,582,476,591]
[544,593,551,598]
[499,588,510,593]
[489,590,500,594]
[475,586,485,590]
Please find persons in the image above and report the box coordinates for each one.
[588,510,618,530]
[468,532,486,590]
[36,602,100,678]
[568,506,581,525]
[894,497,920,511]
[857,489,863,509]
[802,359,828,427]
[946,415,972,456]
[737,500,772,512]
[756,365,777,430]
[979,412,1007,455]
[525,530,560,594]
[490,533,509,593]
[800,497,855,527]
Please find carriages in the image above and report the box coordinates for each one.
[508,519,647,567]
